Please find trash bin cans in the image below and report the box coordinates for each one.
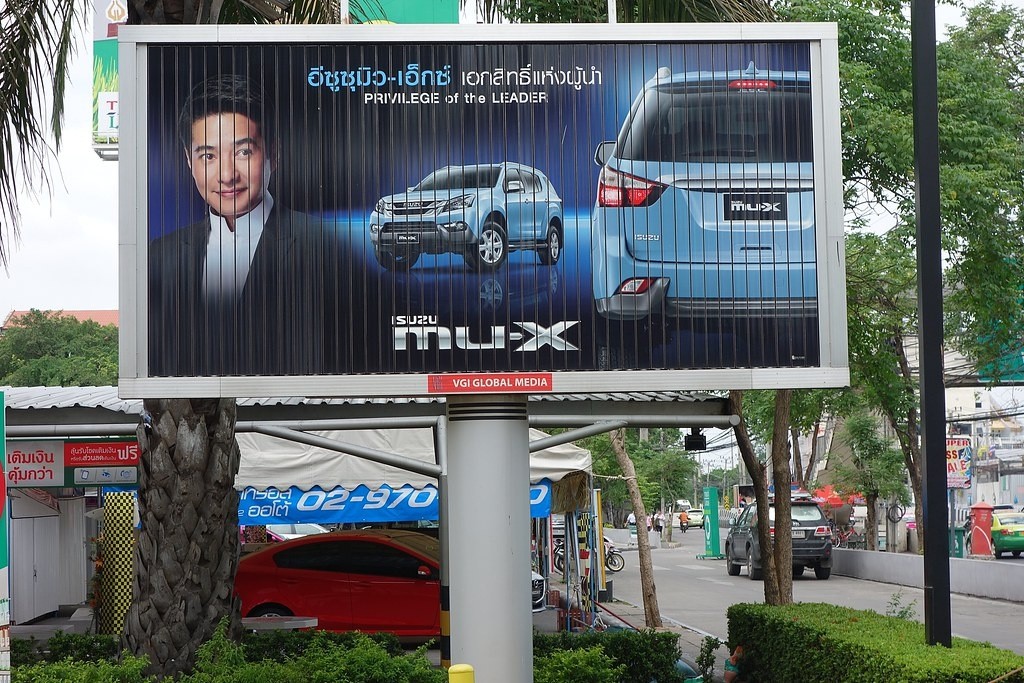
[948,526,966,557]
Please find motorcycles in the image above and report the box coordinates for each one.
[677,516,691,533]
[552,534,624,572]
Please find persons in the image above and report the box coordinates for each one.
[646,511,665,542]
[624,510,636,528]
[148,74,367,377]
[679,508,688,530]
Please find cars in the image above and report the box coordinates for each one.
[790,490,813,506]
[686,509,705,530]
[234,529,548,638]
[263,524,330,538]
[959,510,1024,559]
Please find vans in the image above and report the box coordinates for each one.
[675,500,692,512]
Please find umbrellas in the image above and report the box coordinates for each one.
[797,484,868,546]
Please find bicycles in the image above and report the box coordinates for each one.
[830,521,858,548]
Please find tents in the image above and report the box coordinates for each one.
[234,425,591,636]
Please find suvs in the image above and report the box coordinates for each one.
[369,161,565,271]
[590,67,815,355]
[725,498,834,577]
